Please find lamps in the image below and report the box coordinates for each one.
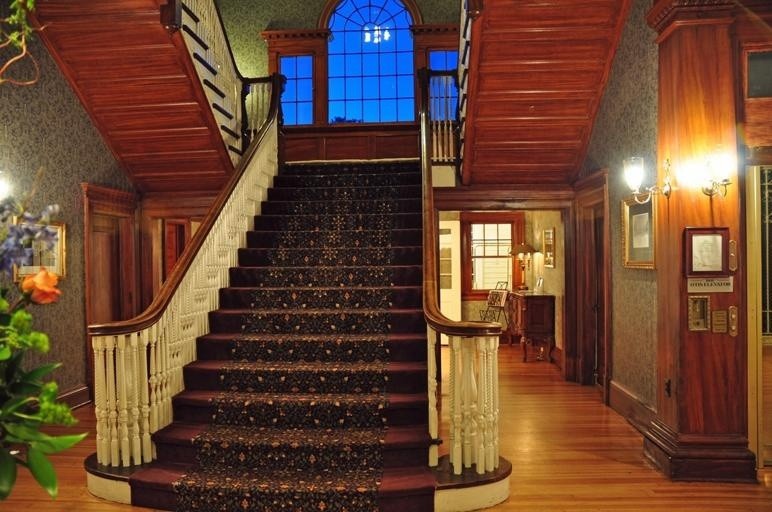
[701,149,738,201]
[509,240,540,293]
[621,156,675,211]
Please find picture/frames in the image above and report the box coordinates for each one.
[11,213,68,285]
[620,191,656,274]
[682,225,729,282]
[539,225,558,270]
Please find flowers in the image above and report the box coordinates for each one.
[0,164,93,510]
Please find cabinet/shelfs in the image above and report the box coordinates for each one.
[507,288,556,363]
